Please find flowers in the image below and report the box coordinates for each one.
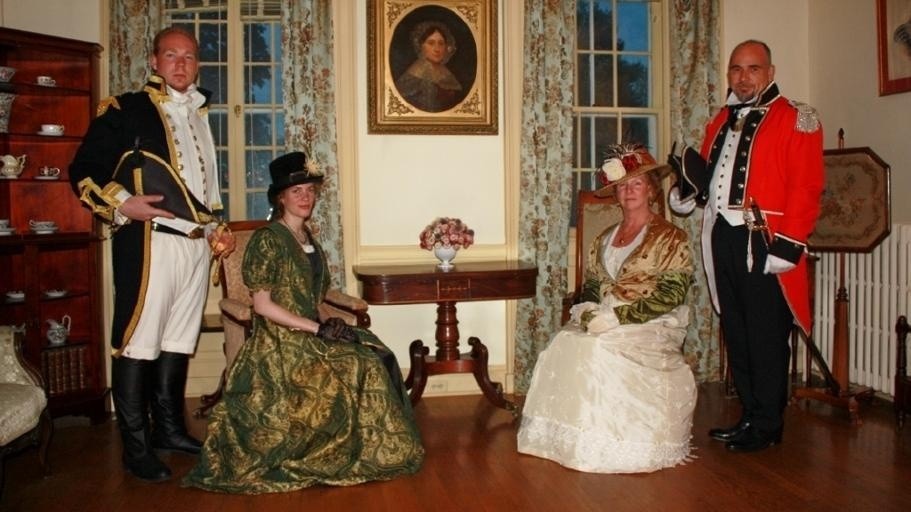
[417,214,476,251]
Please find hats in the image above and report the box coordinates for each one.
[592,149,672,196]
[268,150,324,204]
[668,139,712,208]
[115,150,213,225]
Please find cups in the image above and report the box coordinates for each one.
[40,124,65,134]
[39,166,60,177]
[0,219,10,232]
[37,76,56,87]
[0,92,18,133]
[29,219,55,228]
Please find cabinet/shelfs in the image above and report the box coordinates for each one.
[1,26,112,428]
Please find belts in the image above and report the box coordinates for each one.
[152,215,207,239]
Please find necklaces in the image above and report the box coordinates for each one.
[619,224,634,244]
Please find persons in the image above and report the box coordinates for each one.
[180,152,425,495]
[67,26,236,483]
[667,39,825,454]
[394,20,464,112]
[517,142,698,473]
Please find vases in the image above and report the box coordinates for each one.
[435,246,458,271]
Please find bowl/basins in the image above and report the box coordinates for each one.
[0,66,19,83]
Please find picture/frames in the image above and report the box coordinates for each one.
[365,1,501,139]
[876,0,911,100]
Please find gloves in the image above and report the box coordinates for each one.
[587,309,620,333]
[315,317,355,342]
[764,254,796,275]
[668,185,697,214]
[571,300,594,322]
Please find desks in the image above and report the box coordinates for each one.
[351,261,540,418]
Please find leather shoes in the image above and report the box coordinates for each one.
[708,421,781,452]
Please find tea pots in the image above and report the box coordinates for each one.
[46,314,73,346]
[0,154,27,179]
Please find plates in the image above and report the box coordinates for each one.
[46,290,65,296]
[33,176,60,181]
[32,227,58,233]
[6,291,25,298]
[0,230,11,236]
[38,131,64,137]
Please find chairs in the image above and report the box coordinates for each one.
[1,319,57,472]
[559,180,671,327]
[190,219,270,421]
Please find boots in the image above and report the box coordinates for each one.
[154,350,204,455]
[111,352,170,482]
